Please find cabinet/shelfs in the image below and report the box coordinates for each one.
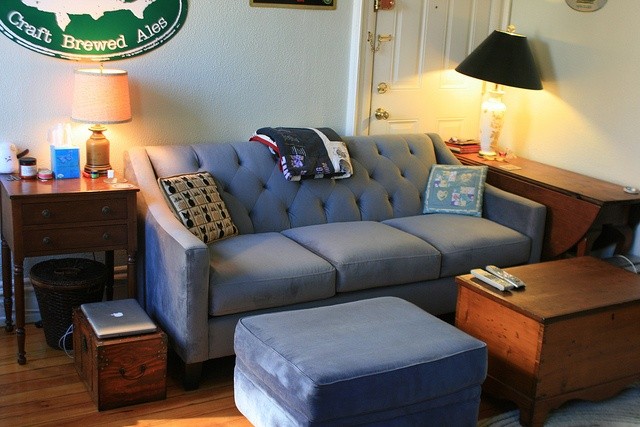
[0,173,140,366]
[71,308,169,412]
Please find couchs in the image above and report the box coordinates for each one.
[131,132,547,391]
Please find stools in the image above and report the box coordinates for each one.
[232,295,489,427]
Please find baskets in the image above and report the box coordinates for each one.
[30,258,106,351]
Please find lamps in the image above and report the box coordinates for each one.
[455,24,543,162]
[70,60,132,179]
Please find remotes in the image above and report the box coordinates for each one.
[487,265,528,291]
[472,265,512,297]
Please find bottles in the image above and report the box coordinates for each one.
[20,157,37,180]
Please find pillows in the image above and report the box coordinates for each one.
[158,171,238,245]
[423,165,488,218]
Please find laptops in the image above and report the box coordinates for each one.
[81,293,160,340]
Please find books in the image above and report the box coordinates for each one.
[445,142,482,154]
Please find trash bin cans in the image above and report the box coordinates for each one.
[28,256,111,359]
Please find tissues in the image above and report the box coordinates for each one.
[49,123,86,182]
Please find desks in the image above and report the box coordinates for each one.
[457,149,640,257]
